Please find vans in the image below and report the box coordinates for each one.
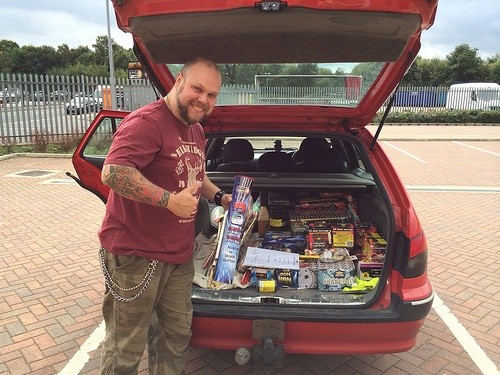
[445,82,500,111]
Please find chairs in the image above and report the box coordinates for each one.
[215,137,348,173]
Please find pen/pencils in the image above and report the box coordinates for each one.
[202,251,214,282]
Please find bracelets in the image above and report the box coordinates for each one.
[214,190,226,206]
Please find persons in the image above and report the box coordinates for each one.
[98,56,252,375]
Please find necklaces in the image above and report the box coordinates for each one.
[167,94,172,111]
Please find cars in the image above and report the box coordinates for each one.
[64,0,439,375]
[0,85,125,108]
[66,96,99,115]
